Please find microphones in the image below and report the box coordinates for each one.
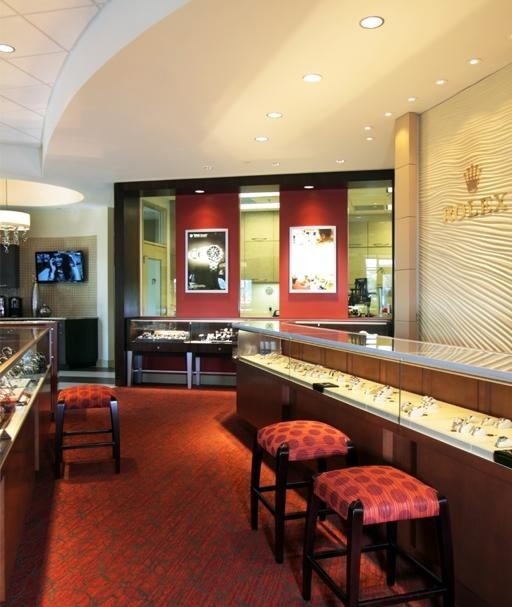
[250,420,355,564]
[303,461,455,607]
[54,384,120,480]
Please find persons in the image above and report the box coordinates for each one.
[48,252,81,281]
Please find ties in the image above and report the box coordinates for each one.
[0,244,19,291]
[35,250,89,284]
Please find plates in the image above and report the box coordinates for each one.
[124,316,238,390]
[232,321,512,607]
[0,321,59,607]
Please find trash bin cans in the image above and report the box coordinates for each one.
[0,346,48,388]
[255,351,512,449]
[206,244,224,272]
[198,327,233,340]
[142,331,185,339]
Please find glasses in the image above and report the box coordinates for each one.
[0,178,31,253]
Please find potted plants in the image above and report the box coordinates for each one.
[185,228,229,294]
[289,226,337,294]
